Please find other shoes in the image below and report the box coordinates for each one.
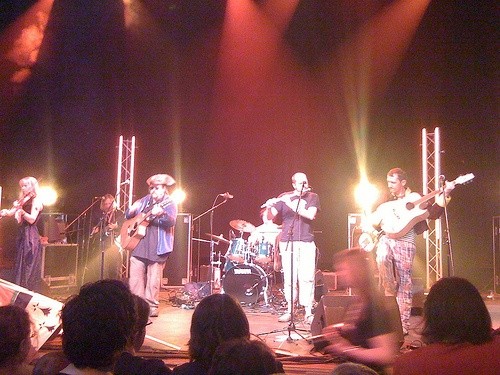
[305,315,314,323]
[150,306,159,317]
[278,313,294,322]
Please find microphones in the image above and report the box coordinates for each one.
[439,175,446,183]
[219,192,234,199]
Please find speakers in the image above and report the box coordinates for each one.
[41,243,79,287]
[320,273,338,291]
[492,217,500,296]
[310,296,359,338]
[410,289,430,315]
[162,214,192,288]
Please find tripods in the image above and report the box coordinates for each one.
[184,200,230,308]
[257,184,309,340]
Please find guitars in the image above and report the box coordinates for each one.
[121,195,177,250]
[381,173,476,238]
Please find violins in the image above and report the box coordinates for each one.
[13,191,36,222]
[104,207,115,236]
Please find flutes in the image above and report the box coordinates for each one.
[260,189,312,209]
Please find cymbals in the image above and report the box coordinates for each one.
[204,232,231,243]
[230,219,255,232]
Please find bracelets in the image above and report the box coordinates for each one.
[344,346,358,353]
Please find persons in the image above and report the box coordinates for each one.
[252,209,282,244]
[174,294,284,375]
[125,175,176,317]
[394,277,500,375]
[0,177,44,290]
[209,338,278,375]
[33,278,174,375]
[367,168,455,334]
[264,171,320,324]
[0,306,34,375]
[320,246,399,375]
[92,194,124,279]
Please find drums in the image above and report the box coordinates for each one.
[255,242,274,263]
[226,238,247,263]
[224,263,268,306]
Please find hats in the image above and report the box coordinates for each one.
[145,174,176,186]
